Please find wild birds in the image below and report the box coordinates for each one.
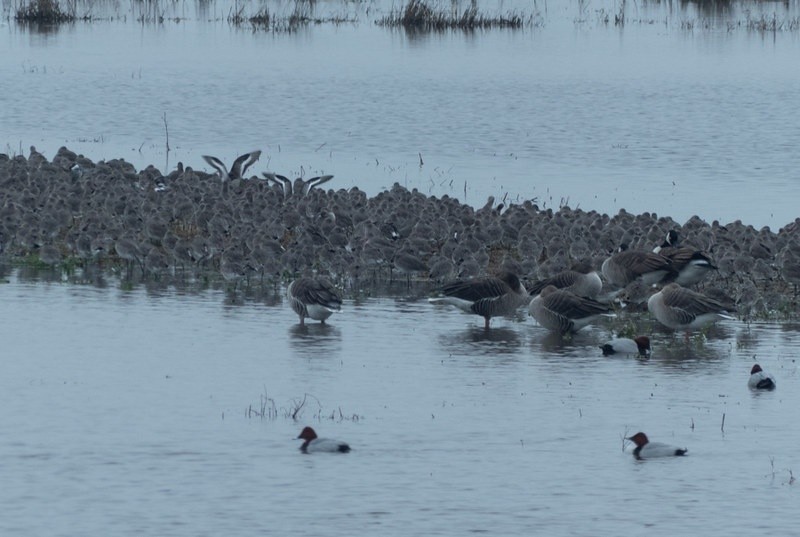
[747,363,777,390]
[293,425,351,453]
[0,146,800,356]
[624,432,688,457]
